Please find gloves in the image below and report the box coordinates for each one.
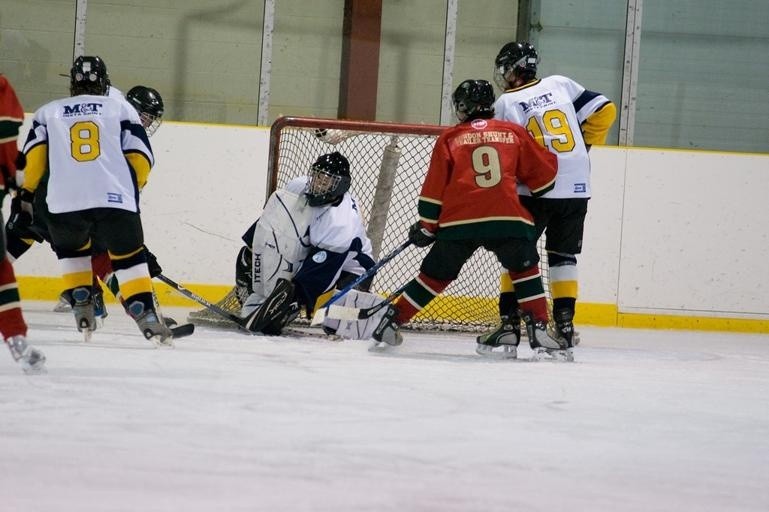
[408,220,436,247]
[12,188,37,225]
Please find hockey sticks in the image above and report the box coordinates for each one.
[326,281,413,321]
[152,284,195,338]
[310,240,413,327]
[157,272,297,333]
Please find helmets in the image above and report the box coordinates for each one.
[304,152,350,206]
[70,55,109,96]
[124,86,164,119]
[453,79,496,122]
[494,42,540,91]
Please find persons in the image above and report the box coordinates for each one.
[0,75,47,373]
[10,56,182,354]
[241,154,375,335]
[63,83,193,341]
[368,78,575,362]
[475,38,617,360]
